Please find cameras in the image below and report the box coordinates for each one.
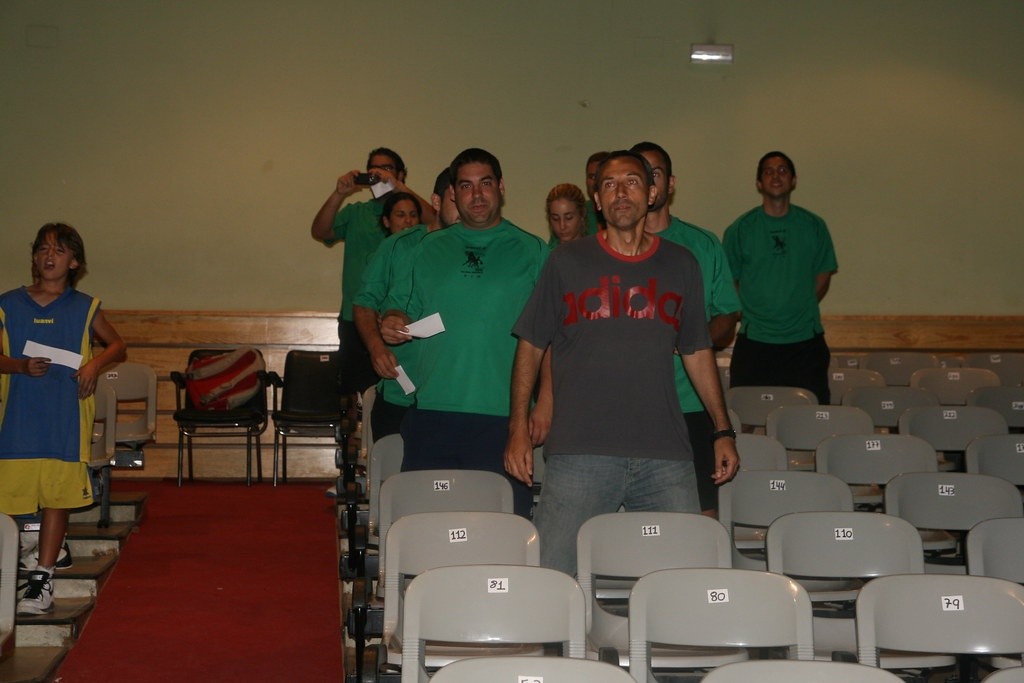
[353,173,378,184]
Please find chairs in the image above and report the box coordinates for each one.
[0,350,1024,683]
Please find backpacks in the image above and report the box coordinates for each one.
[184,346,266,410]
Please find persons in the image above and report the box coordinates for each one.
[311,145,554,520]
[546,151,607,249]
[722,151,840,407]
[631,142,742,520]
[503,150,742,575]
[0,224,126,615]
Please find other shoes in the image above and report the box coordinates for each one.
[325,486,338,496]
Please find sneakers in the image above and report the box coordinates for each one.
[17,541,73,570]
[17,571,55,615]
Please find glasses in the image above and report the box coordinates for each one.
[370,164,397,171]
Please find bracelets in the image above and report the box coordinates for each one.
[712,428,736,441]
